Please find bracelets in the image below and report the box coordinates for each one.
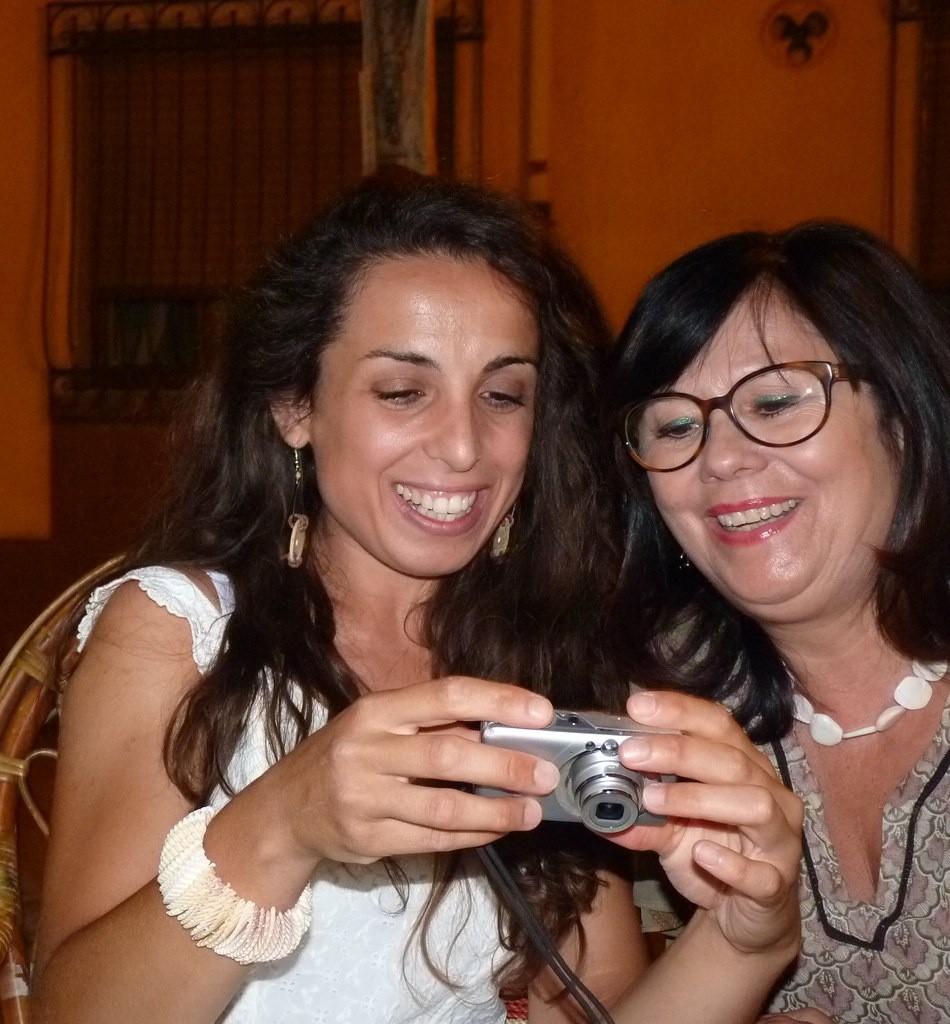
[156,809,313,964]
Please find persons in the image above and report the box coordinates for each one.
[25,160,804,1024]
[614,219,950,1023]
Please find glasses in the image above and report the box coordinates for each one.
[619,359,879,472]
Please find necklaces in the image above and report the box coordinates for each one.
[780,657,947,747]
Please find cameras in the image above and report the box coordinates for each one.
[480,709,684,834]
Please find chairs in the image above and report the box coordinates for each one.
[0,548,131,1024]
